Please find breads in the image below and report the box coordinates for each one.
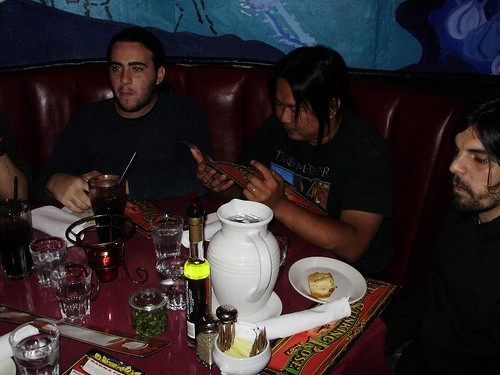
[308,272,335,298]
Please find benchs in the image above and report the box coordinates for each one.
[0,64,458,355]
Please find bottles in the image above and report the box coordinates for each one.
[196,304,238,369]
[184,202,211,348]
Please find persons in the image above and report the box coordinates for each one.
[32,27,210,213]
[190,45,393,281]
[0,113,29,204]
[381,86,500,375]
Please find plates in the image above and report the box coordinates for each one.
[209,286,282,323]
[288,256,367,305]
[204,211,222,241]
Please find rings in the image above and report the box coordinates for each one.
[251,187,256,192]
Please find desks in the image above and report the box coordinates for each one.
[0,196,395,375]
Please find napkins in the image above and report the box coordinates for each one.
[0,332,16,374]
[31,205,86,247]
[257,296,352,341]
[182,213,222,248]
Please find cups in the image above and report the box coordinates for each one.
[129,212,188,337]
[213,321,271,374]
[0,198,92,321]
[8,318,61,375]
[88,174,127,241]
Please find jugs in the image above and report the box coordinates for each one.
[205,198,281,315]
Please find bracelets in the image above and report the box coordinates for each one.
[201,178,209,184]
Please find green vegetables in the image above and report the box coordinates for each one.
[131,302,168,336]
